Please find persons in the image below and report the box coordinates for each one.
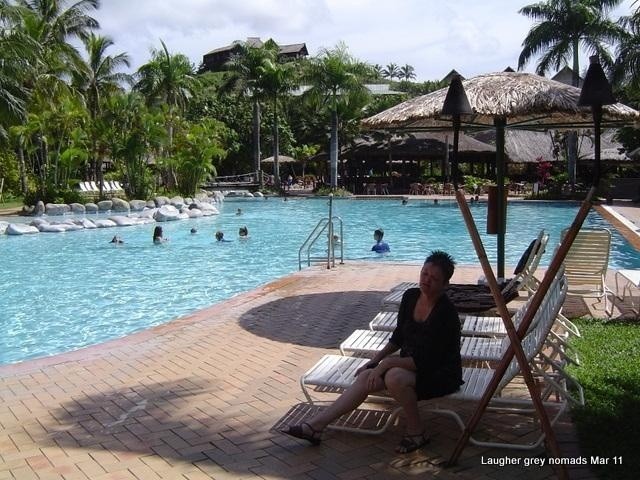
[109,233,127,244]
[189,226,200,235]
[371,227,391,254]
[238,225,250,239]
[432,198,441,206]
[369,167,374,177]
[400,198,409,205]
[287,174,293,184]
[278,249,464,455]
[475,195,479,202]
[212,230,235,242]
[152,224,171,245]
[234,206,246,217]
[469,197,474,204]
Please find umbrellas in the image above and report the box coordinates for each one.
[356,68,639,285]
[260,151,300,164]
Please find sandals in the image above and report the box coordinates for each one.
[280,422,322,445]
[395,430,430,453]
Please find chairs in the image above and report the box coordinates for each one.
[613,238,640,323]
[268,228,589,452]
[365,176,535,199]
[555,223,618,319]
